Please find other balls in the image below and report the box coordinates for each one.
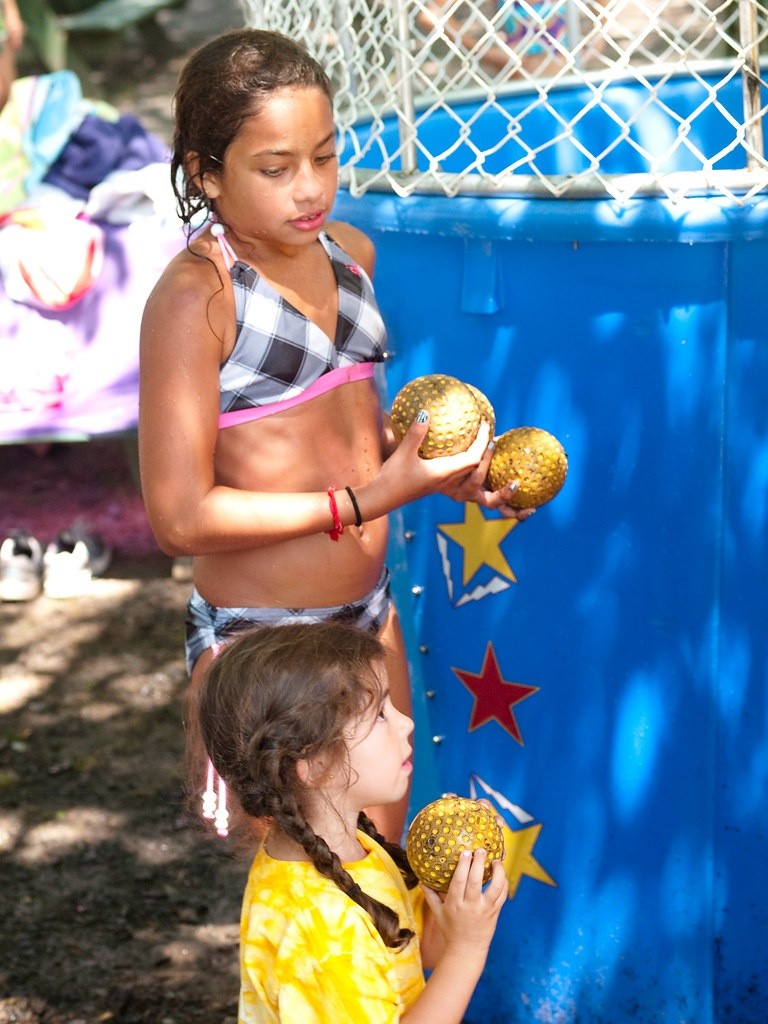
[406,796,505,894]
[391,374,496,460]
[487,427,568,510]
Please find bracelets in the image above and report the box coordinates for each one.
[326,484,343,543]
[346,486,362,527]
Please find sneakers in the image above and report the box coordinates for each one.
[0,535,47,601]
[43,528,111,599]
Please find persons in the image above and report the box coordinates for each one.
[137,32,539,848]
[198,623,508,1024]
